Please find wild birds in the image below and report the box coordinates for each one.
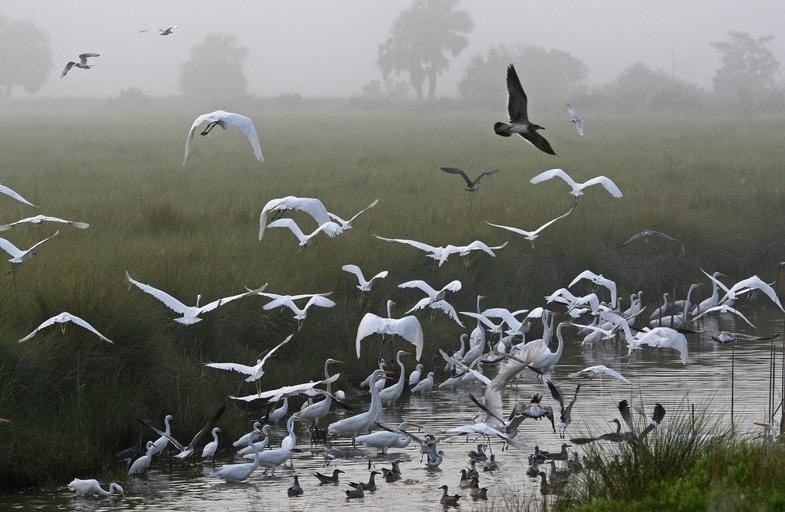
[60,53,100,77]
[0,230,59,275]
[183,110,265,166]
[622,224,674,253]
[19,312,114,344]
[530,169,623,205]
[0,184,37,208]
[567,104,585,136]
[139,26,177,35]
[440,167,498,199]
[124,265,781,502]
[256,197,572,265]
[67,477,124,497]
[0,215,90,231]
[494,64,557,156]
